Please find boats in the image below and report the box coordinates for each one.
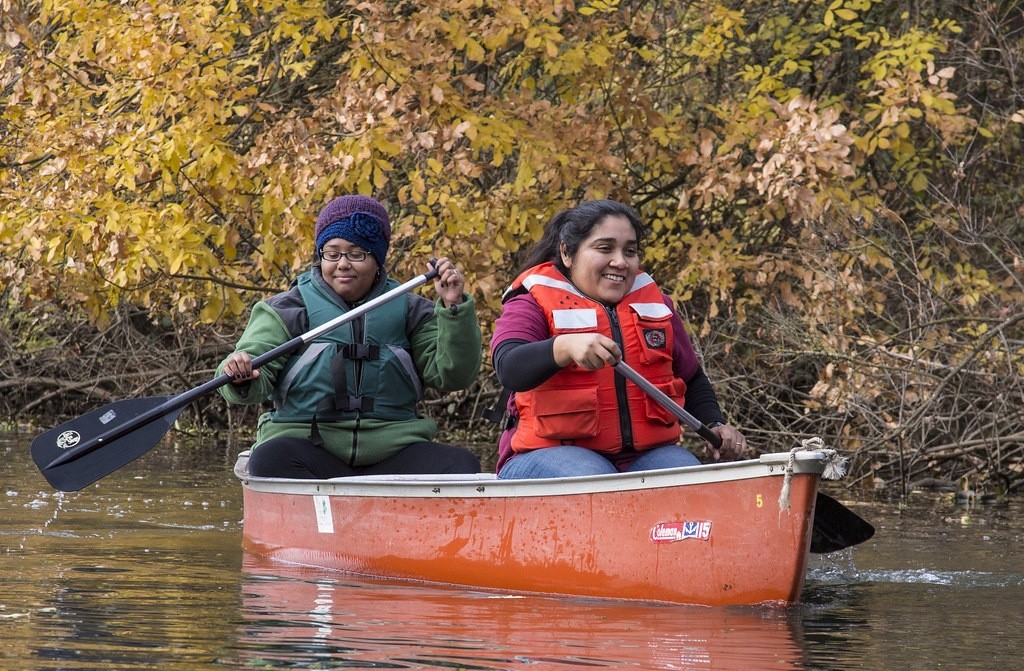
[231,440,832,609]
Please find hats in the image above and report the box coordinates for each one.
[314,195,391,268]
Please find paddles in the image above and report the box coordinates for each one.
[30,260,445,493]
[615,358,877,555]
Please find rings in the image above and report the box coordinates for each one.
[737,442,742,447]
[452,269,457,274]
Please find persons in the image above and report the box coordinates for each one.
[213,194,486,479]
[486,199,749,479]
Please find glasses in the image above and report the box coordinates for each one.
[319,248,372,262]
